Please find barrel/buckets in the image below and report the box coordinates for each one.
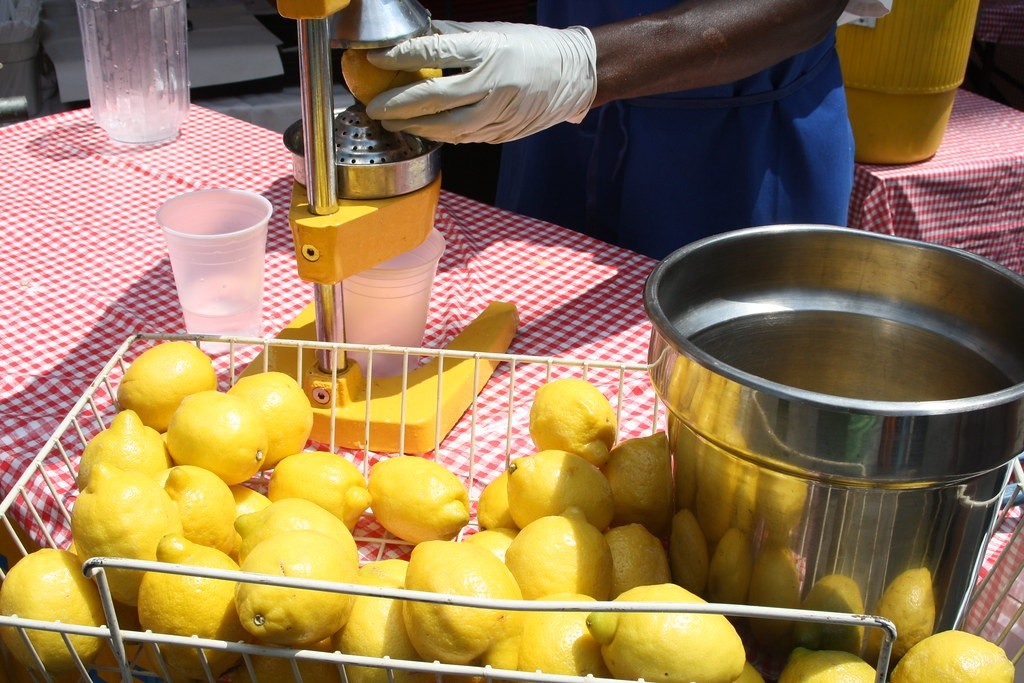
[645,225,1024,683]
[837,0,979,165]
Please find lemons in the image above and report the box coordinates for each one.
[0,341,1022,683]
[342,48,443,106]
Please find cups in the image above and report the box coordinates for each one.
[342,229,446,378]
[77,0,190,144]
[157,190,273,351]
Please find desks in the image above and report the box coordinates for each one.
[0,93,1024,683]
[845,0,1024,279]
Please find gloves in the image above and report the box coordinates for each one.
[364,18,598,145]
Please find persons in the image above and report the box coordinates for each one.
[364,0,857,263]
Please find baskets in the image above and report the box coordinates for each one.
[0,332,1024,682]
